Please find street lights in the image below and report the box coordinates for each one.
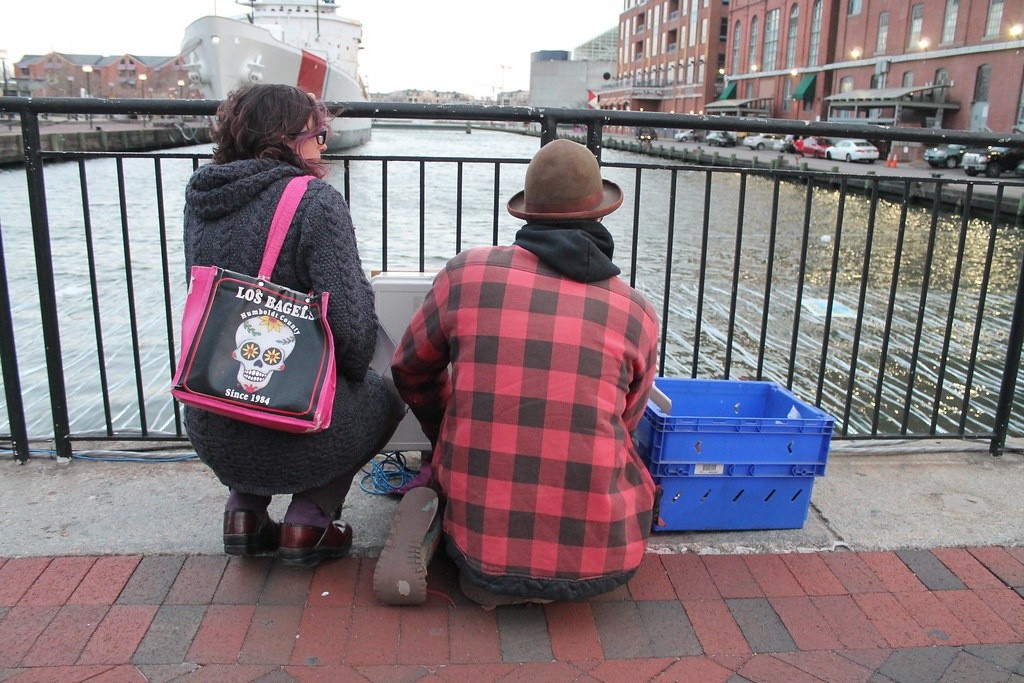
[82,64,94,128]
[68,77,73,96]
[178,81,184,99]
[138,74,148,127]
[0,50,8,96]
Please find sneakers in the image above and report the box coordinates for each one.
[372,487,456,607]
[459,571,555,611]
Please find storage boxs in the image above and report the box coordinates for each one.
[629,377,834,531]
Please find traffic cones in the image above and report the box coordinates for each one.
[891,153,897,168]
[886,153,892,167]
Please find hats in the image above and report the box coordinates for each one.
[506,139,624,218]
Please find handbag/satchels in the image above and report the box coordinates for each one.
[170,175,336,432]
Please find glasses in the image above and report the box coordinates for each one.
[597,216,603,223]
[296,130,327,145]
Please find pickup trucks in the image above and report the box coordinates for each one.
[773,134,798,153]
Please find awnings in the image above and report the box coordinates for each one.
[719,82,737,100]
[791,74,817,100]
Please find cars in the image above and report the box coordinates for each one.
[824,139,880,163]
[675,129,700,142]
[703,131,737,147]
[924,143,977,168]
[800,136,834,158]
[743,133,786,152]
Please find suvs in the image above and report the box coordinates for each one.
[963,147,1024,178]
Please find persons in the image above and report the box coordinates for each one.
[372,138,658,606]
[171,85,406,567]
[792,135,805,165]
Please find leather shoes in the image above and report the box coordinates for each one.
[279,520,354,567]
[223,510,284,556]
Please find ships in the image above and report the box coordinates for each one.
[176,0,374,154]
